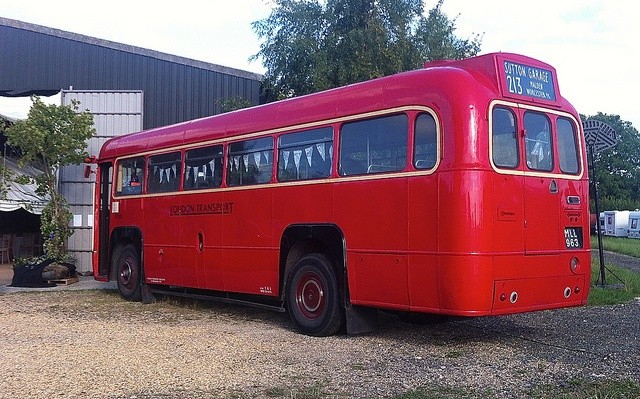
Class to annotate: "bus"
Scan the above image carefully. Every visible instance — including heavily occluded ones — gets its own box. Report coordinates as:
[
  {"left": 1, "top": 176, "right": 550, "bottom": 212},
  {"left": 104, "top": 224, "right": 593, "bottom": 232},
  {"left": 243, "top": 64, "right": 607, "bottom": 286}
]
[{"left": 83, "top": 51, "right": 592, "bottom": 336}]
